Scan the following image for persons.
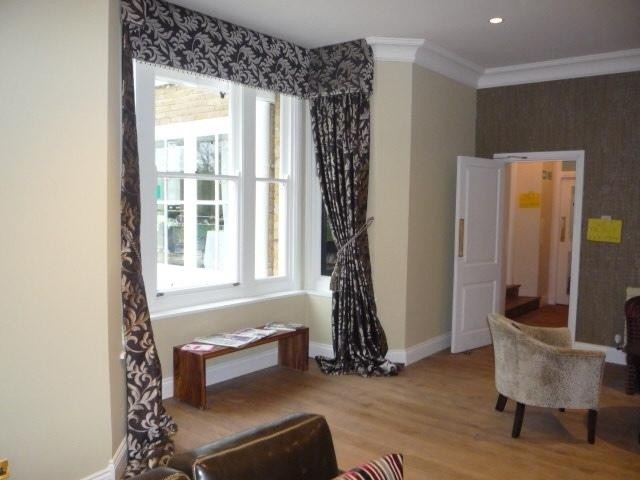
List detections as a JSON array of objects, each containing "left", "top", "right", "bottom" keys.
[{"left": 182, "top": 344, "right": 214, "bottom": 352}]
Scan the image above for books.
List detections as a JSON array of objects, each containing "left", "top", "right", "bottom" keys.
[{"left": 264, "top": 321, "right": 303, "bottom": 332}]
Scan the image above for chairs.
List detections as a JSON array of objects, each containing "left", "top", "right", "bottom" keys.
[{"left": 488, "top": 309, "right": 607, "bottom": 445}]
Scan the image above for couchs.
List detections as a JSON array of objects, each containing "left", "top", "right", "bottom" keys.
[{"left": 130, "top": 413, "right": 342, "bottom": 480}]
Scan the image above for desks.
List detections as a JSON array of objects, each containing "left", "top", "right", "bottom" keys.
[{"left": 176, "top": 324, "right": 311, "bottom": 409}]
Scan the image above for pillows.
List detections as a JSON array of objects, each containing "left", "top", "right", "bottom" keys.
[{"left": 330, "top": 450, "right": 404, "bottom": 480}]
